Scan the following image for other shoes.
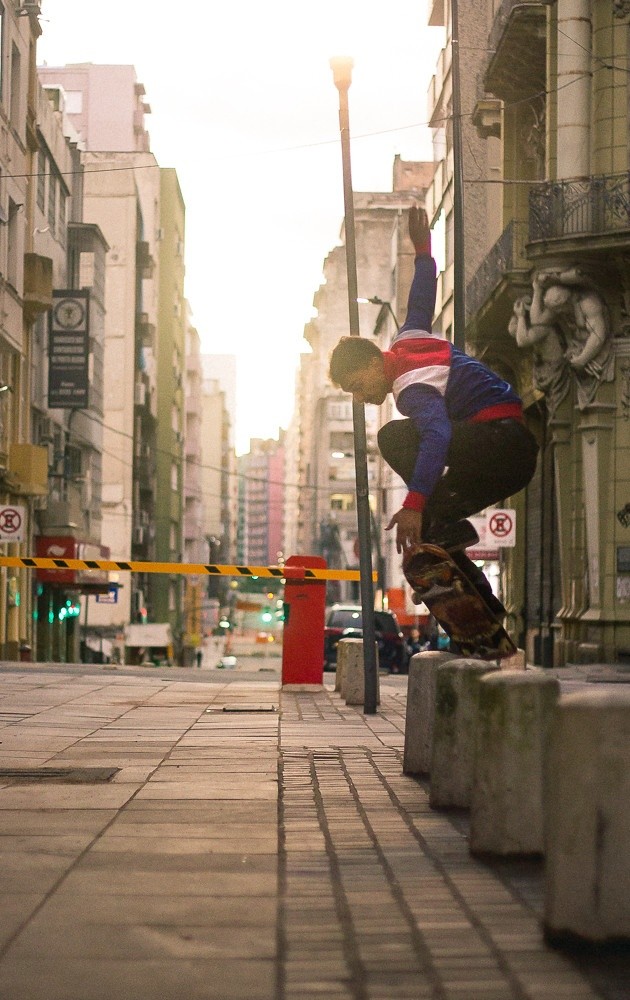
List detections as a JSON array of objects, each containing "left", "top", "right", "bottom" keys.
[
  {"left": 420, "top": 520, "right": 480, "bottom": 553},
  {"left": 483, "top": 594, "right": 507, "bottom": 617}
]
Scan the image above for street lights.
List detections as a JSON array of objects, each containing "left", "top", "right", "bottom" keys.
[{"left": 329, "top": 54, "right": 377, "bottom": 713}]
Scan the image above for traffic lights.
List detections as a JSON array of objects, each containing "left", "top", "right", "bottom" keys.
[{"left": 262, "top": 606, "right": 269, "bottom": 623}]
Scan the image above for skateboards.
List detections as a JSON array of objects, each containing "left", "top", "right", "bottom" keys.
[{"left": 402, "top": 543, "right": 517, "bottom": 661}]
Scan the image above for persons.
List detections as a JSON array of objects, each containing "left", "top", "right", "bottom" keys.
[
  {"left": 509, "top": 272, "right": 614, "bottom": 419},
  {"left": 329, "top": 202, "right": 540, "bottom": 661},
  {"left": 407, "top": 631, "right": 451, "bottom": 674},
  {"left": 196, "top": 651, "right": 202, "bottom": 668}
]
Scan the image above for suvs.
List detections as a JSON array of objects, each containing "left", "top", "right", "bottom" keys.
[{"left": 324, "top": 602, "right": 411, "bottom": 675}]
